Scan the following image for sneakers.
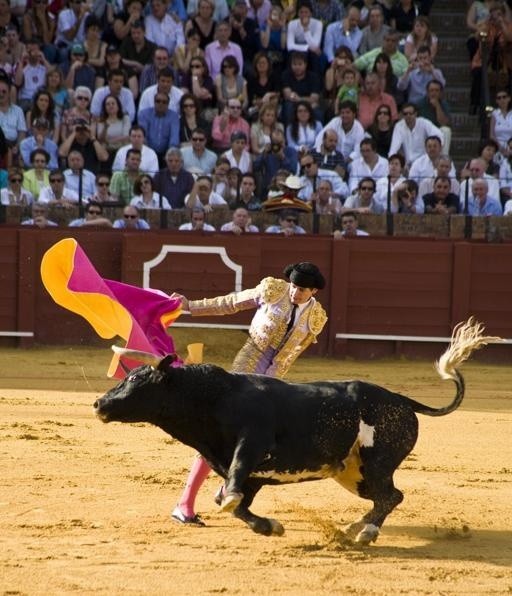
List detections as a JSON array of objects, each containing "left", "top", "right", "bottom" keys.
[
  {"left": 171, "top": 506, "right": 205, "bottom": 527},
  {"left": 215, "top": 487, "right": 224, "bottom": 505},
  {"left": 469, "top": 104, "right": 476, "bottom": 115}
]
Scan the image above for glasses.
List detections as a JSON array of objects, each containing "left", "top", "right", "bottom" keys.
[{"left": 13, "top": 67, "right": 413, "bottom": 217}]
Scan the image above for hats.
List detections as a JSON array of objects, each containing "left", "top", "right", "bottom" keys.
[
  {"left": 284, "top": 262, "right": 327, "bottom": 290},
  {"left": 72, "top": 44, "right": 85, "bottom": 55},
  {"left": 105, "top": 45, "right": 120, "bottom": 53}
]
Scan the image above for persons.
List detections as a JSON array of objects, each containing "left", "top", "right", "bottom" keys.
[
  {"left": 69, "top": 202, "right": 112, "bottom": 226},
  {"left": 113, "top": 206, "right": 149, "bottom": 229},
  {"left": 179, "top": 208, "right": 215, "bottom": 231},
  {"left": 170, "top": 262, "right": 328, "bottom": 527},
  {"left": 265, "top": 211, "right": 305, "bottom": 236},
  {"left": 221, "top": 208, "right": 259, "bottom": 234},
  {"left": 21, "top": 203, "right": 57, "bottom": 228},
  {"left": 334, "top": 212, "right": 368, "bottom": 240},
  {"left": 1, "top": 0, "right": 511, "bottom": 215}
]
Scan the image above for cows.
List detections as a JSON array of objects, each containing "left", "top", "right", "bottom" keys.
[{"left": 93, "top": 315, "right": 504, "bottom": 547}]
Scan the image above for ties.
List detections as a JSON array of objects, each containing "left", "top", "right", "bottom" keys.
[{"left": 286, "top": 303, "right": 299, "bottom": 332}]
